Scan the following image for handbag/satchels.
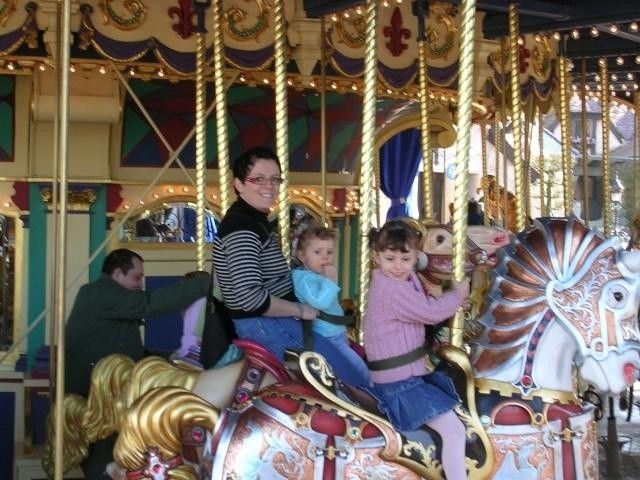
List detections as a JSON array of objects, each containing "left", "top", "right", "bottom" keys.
[{"left": 198, "top": 295, "right": 240, "bottom": 370}]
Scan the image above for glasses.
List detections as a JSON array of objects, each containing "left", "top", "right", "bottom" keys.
[{"left": 244, "top": 176, "right": 284, "bottom": 185}]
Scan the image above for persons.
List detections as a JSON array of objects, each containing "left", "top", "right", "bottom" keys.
[
  {"left": 212, "top": 143, "right": 372, "bottom": 389},
  {"left": 197, "top": 303, "right": 241, "bottom": 366},
  {"left": 56, "top": 249, "right": 209, "bottom": 480},
  {"left": 295, "top": 227, "right": 356, "bottom": 344},
  {"left": 364, "top": 221, "right": 472, "bottom": 479}
]
